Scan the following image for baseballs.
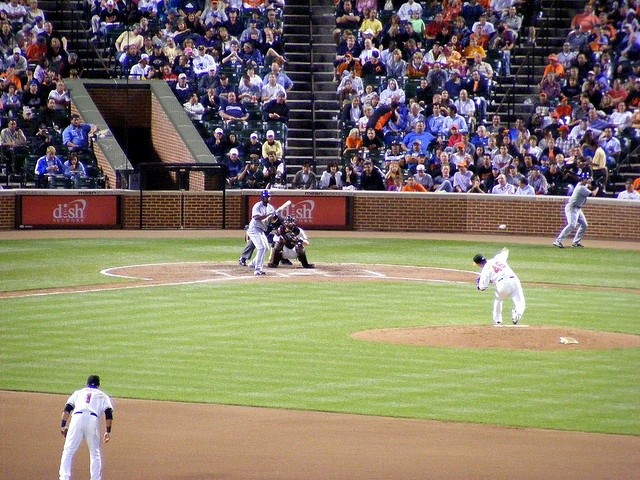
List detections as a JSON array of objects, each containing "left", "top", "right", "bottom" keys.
[{"left": 500, "top": 224, "right": 507, "bottom": 230}]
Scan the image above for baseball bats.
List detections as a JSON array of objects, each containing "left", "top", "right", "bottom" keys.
[{"left": 261, "top": 200, "right": 292, "bottom": 226}]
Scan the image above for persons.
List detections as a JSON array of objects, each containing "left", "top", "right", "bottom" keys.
[
  {"left": 551, "top": 170, "right": 600, "bottom": 249},
  {"left": 320, "top": 1, "right": 572, "bottom": 195},
  {"left": 57, "top": 375, "right": 114, "bottom": 480},
  {"left": 268, "top": 214, "right": 315, "bottom": 270},
  {"left": 246, "top": 190, "right": 276, "bottom": 276},
  {"left": 291, "top": 162, "right": 317, "bottom": 191},
  {"left": 0, "top": 0, "right": 89, "bottom": 188},
  {"left": 89, "top": 0, "right": 289, "bottom": 190},
  {"left": 616, "top": 178, "right": 640, "bottom": 201},
  {"left": 575, "top": 0, "right": 640, "bottom": 177},
  {"left": 238, "top": 209, "right": 293, "bottom": 267},
  {"left": 472, "top": 247, "right": 526, "bottom": 326}
]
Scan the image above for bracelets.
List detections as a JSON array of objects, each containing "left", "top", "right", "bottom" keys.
[
  {"left": 61, "top": 419, "right": 66, "bottom": 427},
  {"left": 106, "top": 426, "right": 111, "bottom": 433}
]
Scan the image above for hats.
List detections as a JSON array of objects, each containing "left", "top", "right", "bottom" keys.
[
  {"left": 138, "top": 53, "right": 149, "bottom": 62},
  {"left": 473, "top": 254, "right": 486, "bottom": 264},
  {"left": 229, "top": 147, "right": 239, "bottom": 155},
  {"left": 547, "top": 106, "right": 556, "bottom": 113},
  {"left": 580, "top": 171, "right": 590, "bottom": 179},
  {"left": 541, "top": 154, "right": 548, "bottom": 161},
  {"left": 457, "top": 162, "right": 466, "bottom": 167},
  {"left": 523, "top": 143, "right": 530, "bottom": 149},
  {"left": 34, "top": 16, "right": 42, "bottom": 22},
  {"left": 215, "top": 127, "right": 224, "bottom": 134},
  {"left": 106, "top": 0, "right": 114, "bottom": 6},
  {"left": 547, "top": 53, "right": 558, "bottom": 62},
  {"left": 391, "top": 140, "right": 399, "bottom": 145},
  {"left": 413, "top": 138, "right": 422, "bottom": 145},
  {"left": 250, "top": 133, "right": 258, "bottom": 139},
  {"left": 29, "top": 80, "right": 37, "bottom": 86},
  {"left": 457, "top": 143, "right": 465, "bottom": 149},
  {"left": 88, "top": 374, "right": 100, "bottom": 386},
  {"left": 556, "top": 125, "right": 570, "bottom": 131},
  {"left": 178, "top": 73, "right": 187, "bottom": 79},
  {"left": 13, "top": 47, "right": 22, "bottom": 54},
  {"left": 586, "top": 70, "right": 595, "bottom": 75},
  {"left": 492, "top": 165, "right": 500, "bottom": 170},
  {"left": 266, "top": 129, "right": 275, "bottom": 137},
  {"left": 450, "top": 124, "right": 459, "bottom": 130},
  {"left": 509, "top": 163, "right": 517, "bottom": 168},
  {"left": 416, "top": 164, "right": 426, "bottom": 171},
  {"left": 417, "top": 151, "right": 426, "bottom": 157}
]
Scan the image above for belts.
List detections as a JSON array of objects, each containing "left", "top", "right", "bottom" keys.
[
  {"left": 573, "top": 205, "right": 581, "bottom": 208},
  {"left": 498, "top": 276, "right": 514, "bottom": 282},
  {"left": 73, "top": 411, "right": 98, "bottom": 417}
]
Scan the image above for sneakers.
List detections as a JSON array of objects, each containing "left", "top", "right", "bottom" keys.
[
  {"left": 512, "top": 309, "right": 518, "bottom": 324},
  {"left": 248, "top": 262, "right": 255, "bottom": 269},
  {"left": 268, "top": 263, "right": 278, "bottom": 268},
  {"left": 303, "top": 263, "right": 315, "bottom": 269},
  {"left": 239, "top": 256, "right": 246, "bottom": 266},
  {"left": 493, "top": 321, "right": 502, "bottom": 325},
  {"left": 254, "top": 269, "right": 267, "bottom": 275},
  {"left": 283, "top": 260, "right": 292, "bottom": 265},
  {"left": 571, "top": 243, "right": 584, "bottom": 247},
  {"left": 553, "top": 241, "right": 564, "bottom": 248}
]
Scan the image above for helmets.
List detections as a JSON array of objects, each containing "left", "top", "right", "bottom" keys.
[
  {"left": 261, "top": 190, "right": 271, "bottom": 198},
  {"left": 272, "top": 215, "right": 283, "bottom": 230},
  {"left": 284, "top": 215, "right": 296, "bottom": 229}
]
